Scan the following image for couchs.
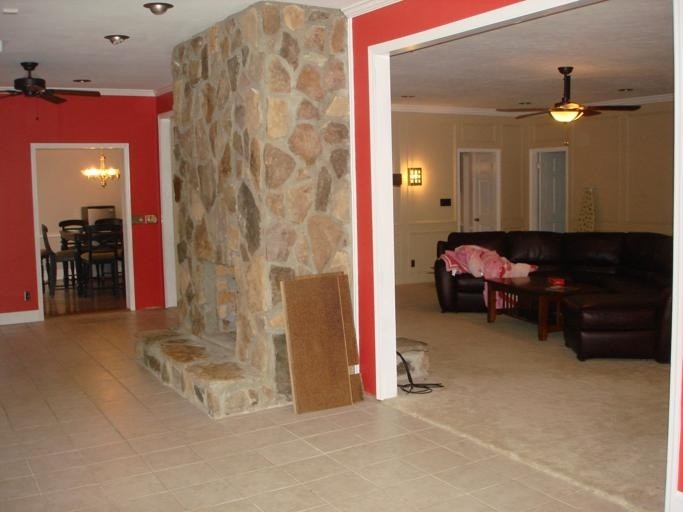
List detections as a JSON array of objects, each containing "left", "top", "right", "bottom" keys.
[{"left": 434, "top": 232, "right": 672, "bottom": 361}]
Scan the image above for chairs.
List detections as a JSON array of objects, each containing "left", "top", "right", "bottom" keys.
[{"left": 42, "top": 217, "right": 125, "bottom": 298}]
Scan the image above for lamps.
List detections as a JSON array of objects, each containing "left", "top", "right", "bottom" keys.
[
  {"left": 104, "top": 35, "right": 130, "bottom": 46},
  {"left": 549, "top": 97, "right": 587, "bottom": 123},
  {"left": 80, "top": 150, "right": 120, "bottom": 193},
  {"left": 143, "top": 2, "right": 174, "bottom": 16},
  {"left": 408, "top": 168, "right": 421, "bottom": 185}
]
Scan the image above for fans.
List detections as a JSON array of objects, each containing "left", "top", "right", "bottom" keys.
[
  {"left": 496, "top": 67, "right": 641, "bottom": 120},
  {"left": 0, "top": 62, "right": 100, "bottom": 104}
]
